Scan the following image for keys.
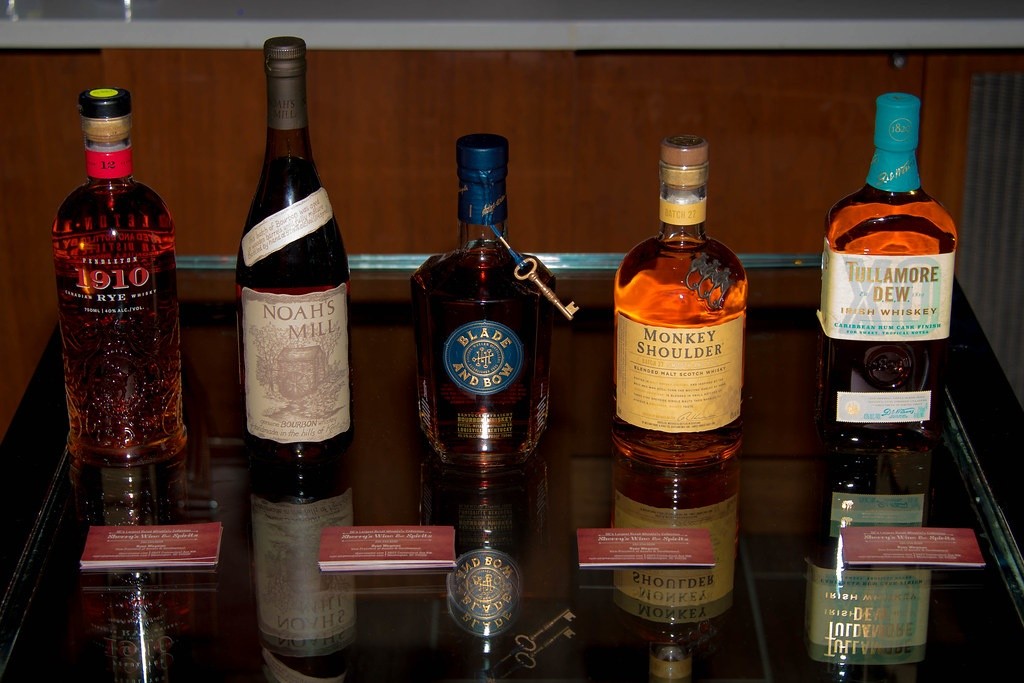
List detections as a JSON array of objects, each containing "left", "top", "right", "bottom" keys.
[
  {"left": 515, "top": 606, "right": 577, "bottom": 669},
  {"left": 514, "top": 257, "right": 579, "bottom": 321}
]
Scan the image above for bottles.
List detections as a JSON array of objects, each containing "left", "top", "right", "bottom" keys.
[
  {"left": 609, "top": 459, "right": 742, "bottom": 683},
  {"left": 804, "top": 446, "right": 935, "bottom": 683},
  {"left": 420, "top": 465, "right": 547, "bottom": 683},
  {"left": 237, "top": 35, "right": 349, "bottom": 464},
  {"left": 50, "top": 88, "right": 187, "bottom": 463},
  {"left": 614, "top": 131, "right": 748, "bottom": 470},
  {"left": 821, "top": 91, "right": 956, "bottom": 448},
  {"left": 71, "top": 465, "right": 193, "bottom": 683},
  {"left": 411, "top": 133, "right": 576, "bottom": 479},
  {"left": 251, "top": 465, "right": 356, "bottom": 683}
]
